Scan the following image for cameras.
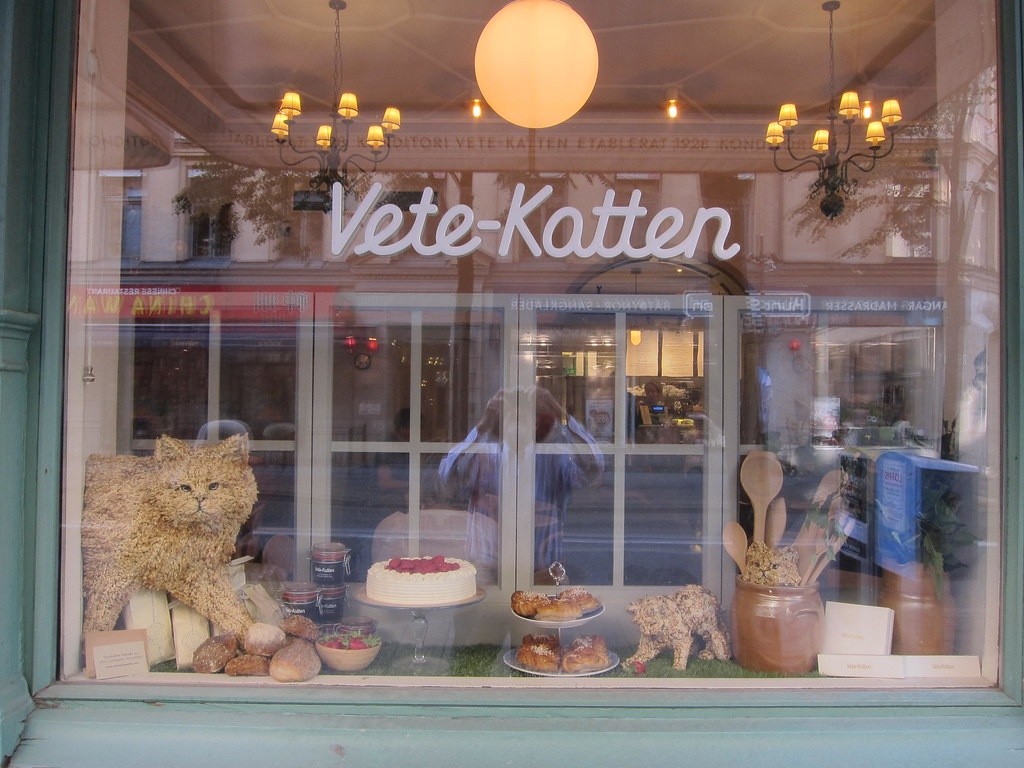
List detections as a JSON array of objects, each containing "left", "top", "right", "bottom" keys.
[{"left": 505, "top": 387, "right": 528, "bottom": 405}]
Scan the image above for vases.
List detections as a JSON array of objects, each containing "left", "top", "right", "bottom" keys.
[{"left": 730, "top": 574, "right": 825, "bottom": 672}]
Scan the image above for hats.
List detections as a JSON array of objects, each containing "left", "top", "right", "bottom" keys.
[{"left": 644, "top": 377, "right": 662, "bottom": 400}]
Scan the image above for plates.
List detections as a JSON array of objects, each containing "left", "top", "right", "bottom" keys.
[
  {"left": 511, "top": 594, "right": 605, "bottom": 628},
  {"left": 502, "top": 645, "right": 619, "bottom": 676}
]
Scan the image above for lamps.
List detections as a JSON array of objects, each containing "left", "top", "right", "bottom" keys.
[
  {"left": 763, "top": 0, "right": 905, "bottom": 219},
  {"left": 630, "top": 331, "right": 641, "bottom": 345},
  {"left": 475, "top": 0, "right": 599, "bottom": 128},
  {"left": 269, "top": 0, "right": 402, "bottom": 198}
]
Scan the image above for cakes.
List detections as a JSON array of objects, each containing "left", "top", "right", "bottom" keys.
[{"left": 366, "top": 556, "right": 477, "bottom": 606}]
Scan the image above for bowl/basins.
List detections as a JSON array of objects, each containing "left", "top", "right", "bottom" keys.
[{"left": 314, "top": 636, "right": 382, "bottom": 672}]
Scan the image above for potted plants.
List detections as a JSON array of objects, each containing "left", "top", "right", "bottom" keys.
[{"left": 872, "top": 472, "right": 984, "bottom": 658}]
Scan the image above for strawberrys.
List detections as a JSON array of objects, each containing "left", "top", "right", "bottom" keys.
[{"left": 319, "top": 633, "right": 373, "bottom": 649}]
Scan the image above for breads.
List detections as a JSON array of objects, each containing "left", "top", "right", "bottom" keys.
[
  {"left": 192, "top": 615, "right": 322, "bottom": 682},
  {"left": 516, "top": 634, "right": 613, "bottom": 673},
  {"left": 511, "top": 589, "right": 602, "bottom": 622}
]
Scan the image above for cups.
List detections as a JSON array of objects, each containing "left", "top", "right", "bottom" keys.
[
  {"left": 724, "top": 578, "right": 824, "bottom": 678},
  {"left": 333, "top": 616, "right": 379, "bottom": 635},
  {"left": 814, "top": 396, "right": 840, "bottom": 444}
]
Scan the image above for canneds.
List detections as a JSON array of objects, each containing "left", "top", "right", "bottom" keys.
[{"left": 275, "top": 542, "right": 378, "bottom": 633}]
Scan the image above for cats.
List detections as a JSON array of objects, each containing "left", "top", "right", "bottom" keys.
[
  {"left": 620, "top": 585, "right": 732, "bottom": 670},
  {"left": 82, "top": 431, "right": 258, "bottom": 641}
]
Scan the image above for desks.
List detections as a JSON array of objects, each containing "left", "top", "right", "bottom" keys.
[{"left": 852, "top": 444, "right": 939, "bottom": 523}]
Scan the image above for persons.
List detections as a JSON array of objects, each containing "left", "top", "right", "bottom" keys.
[
  {"left": 639, "top": 379, "right": 670, "bottom": 421},
  {"left": 370, "top": 411, "right": 428, "bottom": 520},
  {"left": 952, "top": 351, "right": 989, "bottom": 452},
  {"left": 197, "top": 362, "right": 788, "bottom": 705}
]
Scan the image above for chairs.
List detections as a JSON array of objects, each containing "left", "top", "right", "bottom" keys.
[{"left": 343, "top": 424, "right": 373, "bottom": 509}]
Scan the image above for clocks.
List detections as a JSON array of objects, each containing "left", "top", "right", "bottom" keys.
[{"left": 354, "top": 352, "right": 371, "bottom": 370}]
